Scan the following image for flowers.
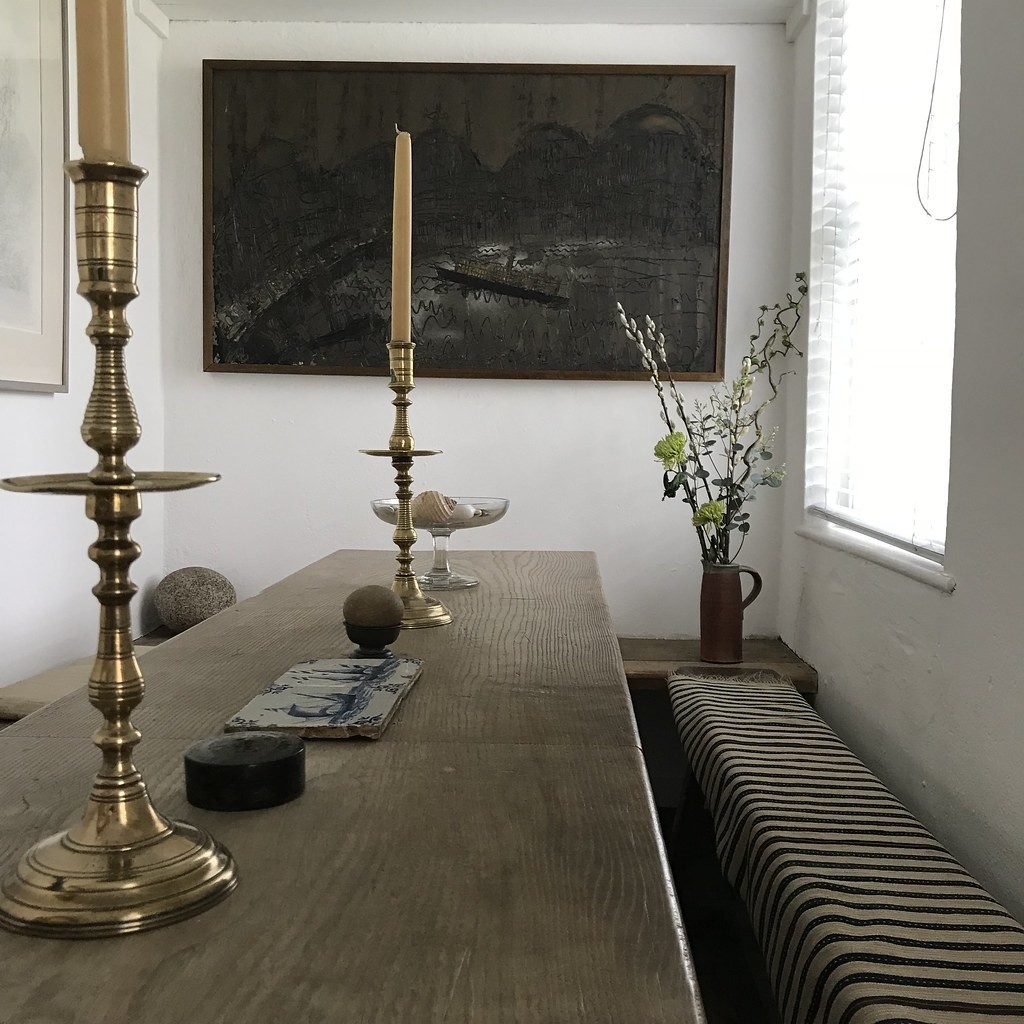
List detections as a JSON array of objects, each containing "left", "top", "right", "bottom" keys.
[{"left": 616, "top": 273, "right": 809, "bottom": 566}]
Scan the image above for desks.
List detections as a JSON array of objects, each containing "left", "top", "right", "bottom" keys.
[{"left": 0, "top": 548, "right": 704, "bottom": 1024}]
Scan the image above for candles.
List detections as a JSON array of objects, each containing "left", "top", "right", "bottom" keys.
[
  {"left": 74, "top": 0, "right": 131, "bottom": 162},
  {"left": 388, "top": 122, "right": 411, "bottom": 343}
]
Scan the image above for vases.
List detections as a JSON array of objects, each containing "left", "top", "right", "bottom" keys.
[{"left": 700, "top": 563, "right": 763, "bottom": 666}]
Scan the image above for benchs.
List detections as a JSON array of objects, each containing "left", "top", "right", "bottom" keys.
[
  {"left": 0, "top": 627, "right": 181, "bottom": 729},
  {"left": 667, "top": 663, "right": 1024, "bottom": 1024},
  {"left": 618, "top": 638, "right": 821, "bottom": 692}
]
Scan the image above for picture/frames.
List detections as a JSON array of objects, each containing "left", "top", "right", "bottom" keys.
[
  {"left": 201, "top": 60, "right": 735, "bottom": 383},
  {"left": 0, "top": 0, "right": 70, "bottom": 393}
]
